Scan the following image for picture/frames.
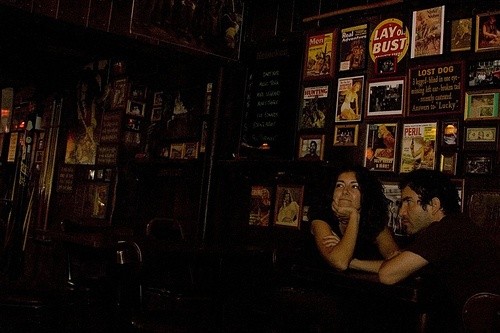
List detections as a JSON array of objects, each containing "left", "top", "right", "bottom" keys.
[
  {"left": 121, "top": 83, "right": 199, "bottom": 160},
  {"left": 272, "top": 3, "right": 500, "bottom": 241}
]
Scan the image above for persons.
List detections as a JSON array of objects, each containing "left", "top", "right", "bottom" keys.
[
  {"left": 306, "top": 161, "right": 401, "bottom": 333},
  {"left": 253, "top": 15, "right": 500, "bottom": 236},
  {"left": 379, "top": 169, "right": 500, "bottom": 333}
]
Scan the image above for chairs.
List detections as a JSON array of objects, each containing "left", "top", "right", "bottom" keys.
[{"left": 0, "top": 197, "right": 215, "bottom": 333}]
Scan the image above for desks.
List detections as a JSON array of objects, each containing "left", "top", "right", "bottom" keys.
[
  {"left": 31, "top": 227, "right": 260, "bottom": 333},
  {"left": 289, "top": 263, "right": 445, "bottom": 333}
]
